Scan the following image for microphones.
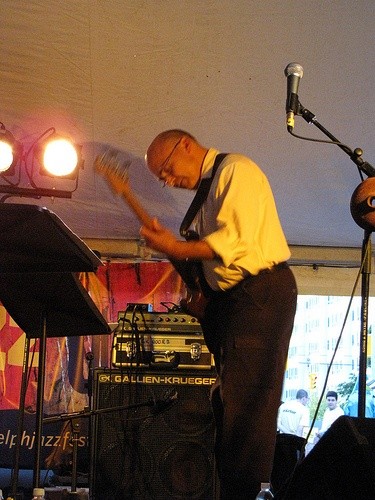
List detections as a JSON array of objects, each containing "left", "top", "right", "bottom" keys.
[{"left": 283, "top": 61, "right": 305, "bottom": 133}]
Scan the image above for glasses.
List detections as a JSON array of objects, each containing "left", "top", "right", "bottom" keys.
[{"left": 157, "top": 137, "right": 182, "bottom": 188}]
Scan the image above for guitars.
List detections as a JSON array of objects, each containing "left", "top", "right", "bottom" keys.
[{"left": 94, "top": 145, "right": 230, "bottom": 367}]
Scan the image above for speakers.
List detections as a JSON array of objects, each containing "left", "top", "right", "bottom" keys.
[
  {"left": 277, "top": 416, "right": 375, "bottom": 500},
  {"left": 90, "top": 367, "right": 219, "bottom": 500}
]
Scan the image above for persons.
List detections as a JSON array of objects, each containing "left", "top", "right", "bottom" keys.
[
  {"left": 314, "top": 391, "right": 344, "bottom": 442},
  {"left": 140, "top": 130, "right": 297, "bottom": 500},
  {"left": 276, "top": 389, "right": 310, "bottom": 439}
]
